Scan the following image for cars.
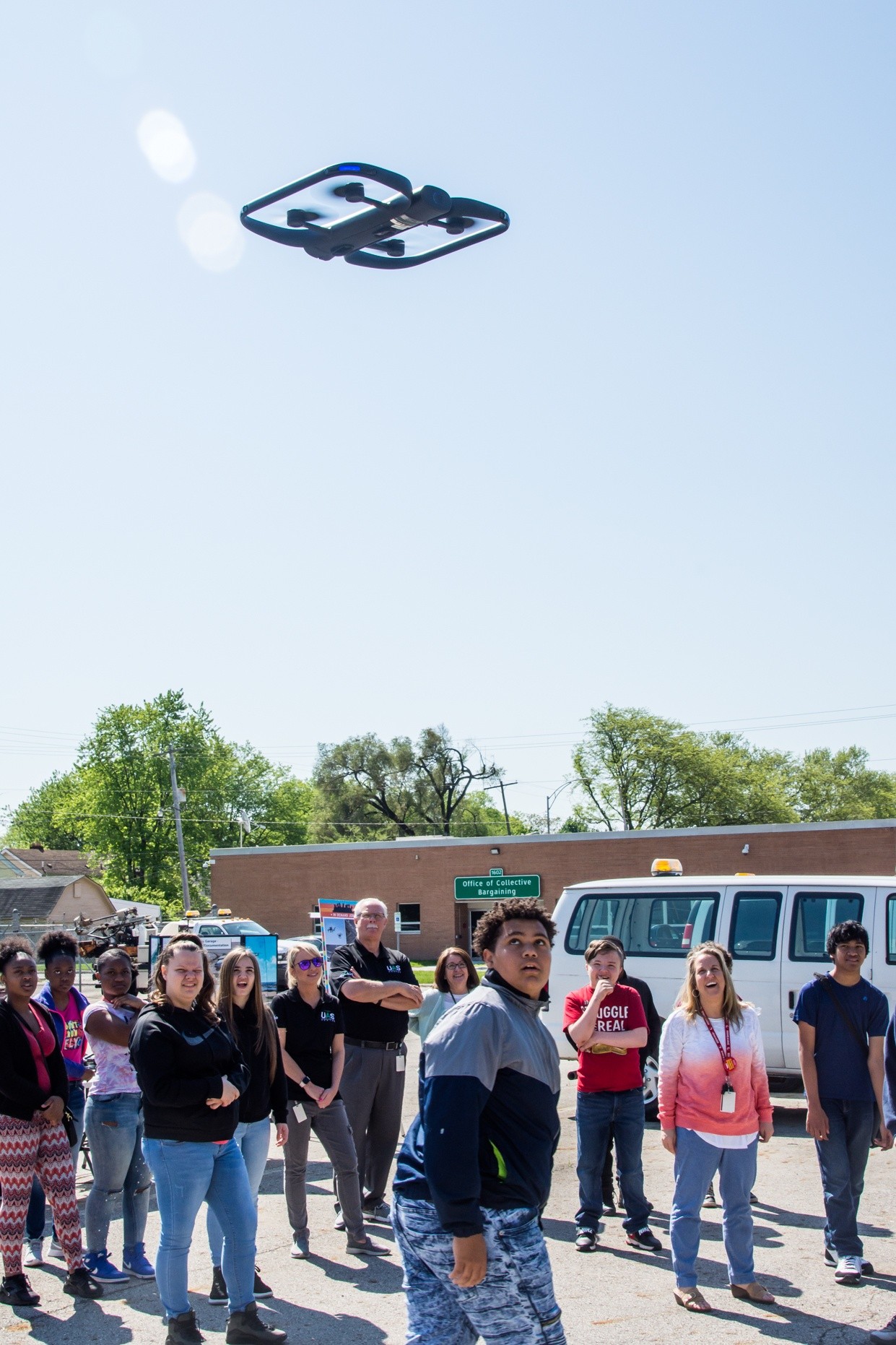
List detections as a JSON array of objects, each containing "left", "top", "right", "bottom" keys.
[{"left": 284, "top": 934, "right": 340, "bottom": 951}]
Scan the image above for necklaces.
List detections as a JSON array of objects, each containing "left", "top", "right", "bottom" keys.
[{"left": 449, "top": 989, "right": 470, "bottom": 1004}]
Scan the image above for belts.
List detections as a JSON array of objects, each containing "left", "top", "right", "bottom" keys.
[{"left": 344, "top": 1038, "right": 404, "bottom": 1050}]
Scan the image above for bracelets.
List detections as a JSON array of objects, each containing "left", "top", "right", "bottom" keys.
[{"left": 376, "top": 999, "right": 382, "bottom": 1006}]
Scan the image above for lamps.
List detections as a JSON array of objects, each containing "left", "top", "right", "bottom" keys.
[{"left": 491, "top": 848, "right": 499, "bottom": 854}]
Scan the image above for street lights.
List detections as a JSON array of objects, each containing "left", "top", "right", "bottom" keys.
[{"left": 546, "top": 776, "right": 589, "bottom": 834}]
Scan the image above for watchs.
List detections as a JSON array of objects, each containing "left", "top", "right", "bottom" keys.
[{"left": 299, "top": 1076, "right": 311, "bottom": 1088}]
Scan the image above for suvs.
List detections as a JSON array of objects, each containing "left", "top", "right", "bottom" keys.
[{"left": 159, "top": 909, "right": 324, "bottom": 991}]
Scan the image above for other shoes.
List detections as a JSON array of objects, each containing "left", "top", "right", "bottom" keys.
[
  {"left": 253, "top": 1264, "right": 273, "bottom": 1297},
  {"left": 870, "top": 1315, "right": 896, "bottom": 1345},
  {"left": 601, "top": 1191, "right": 618, "bottom": 1216},
  {"left": 346, "top": 1237, "right": 391, "bottom": 1255},
  {"left": 208, "top": 1266, "right": 228, "bottom": 1304},
  {"left": 362, "top": 1202, "right": 392, "bottom": 1222},
  {"left": 702, "top": 1182, "right": 717, "bottom": 1207},
  {"left": 618, "top": 1194, "right": 654, "bottom": 1209},
  {"left": 750, "top": 1192, "right": 758, "bottom": 1203},
  {"left": 334, "top": 1210, "right": 345, "bottom": 1229},
  {"left": 290, "top": 1237, "right": 310, "bottom": 1258}
]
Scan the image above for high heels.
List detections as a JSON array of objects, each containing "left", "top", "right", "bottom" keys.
[
  {"left": 730, "top": 1282, "right": 775, "bottom": 1304},
  {"left": 673, "top": 1287, "right": 712, "bottom": 1312}
]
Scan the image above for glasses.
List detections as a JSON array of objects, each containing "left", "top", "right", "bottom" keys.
[
  {"left": 292, "top": 957, "right": 323, "bottom": 971},
  {"left": 357, "top": 912, "right": 384, "bottom": 918},
  {"left": 446, "top": 961, "right": 465, "bottom": 968}
]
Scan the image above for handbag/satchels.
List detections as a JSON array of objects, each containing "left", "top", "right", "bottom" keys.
[{"left": 62, "top": 1108, "right": 79, "bottom": 1147}]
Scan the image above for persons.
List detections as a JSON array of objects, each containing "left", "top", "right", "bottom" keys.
[
  {"left": 656, "top": 941, "right": 776, "bottom": 1312},
  {"left": 270, "top": 943, "right": 391, "bottom": 1258},
  {"left": 206, "top": 945, "right": 289, "bottom": 1305},
  {"left": 407, "top": 947, "right": 480, "bottom": 1050},
  {"left": 328, "top": 898, "right": 424, "bottom": 1230},
  {"left": 389, "top": 898, "right": 568, "bottom": 1345},
  {"left": 127, "top": 933, "right": 288, "bottom": 1345},
  {"left": 870, "top": 1316, "right": 896, "bottom": 1345},
  {"left": 793, "top": 919, "right": 896, "bottom": 1283},
  {"left": 0, "top": 931, "right": 156, "bottom": 1306},
  {"left": 562, "top": 935, "right": 662, "bottom": 1251}
]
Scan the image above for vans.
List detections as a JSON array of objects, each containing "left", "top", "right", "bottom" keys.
[{"left": 549, "top": 857, "right": 896, "bottom": 1122}]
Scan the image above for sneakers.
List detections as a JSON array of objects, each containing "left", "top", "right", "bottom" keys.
[
  {"left": 0, "top": 1273, "right": 40, "bottom": 1305},
  {"left": 23, "top": 1234, "right": 45, "bottom": 1266},
  {"left": 576, "top": 1226, "right": 597, "bottom": 1251},
  {"left": 83, "top": 1249, "right": 131, "bottom": 1284},
  {"left": 165, "top": 1308, "right": 203, "bottom": 1345},
  {"left": 122, "top": 1242, "right": 155, "bottom": 1279},
  {"left": 225, "top": 1300, "right": 287, "bottom": 1343},
  {"left": 62, "top": 1267, "right": 104, "bottom": 1298},
  {"left": 626, "top": 1226, "right": 662, "bottom": 1250},
  {"left": 835, "top": 1255, "right": 862, "bottom": 1283},
  {"left": 824, "top": 1247, "right": 874, "bottom": 1275},
  {"left": 47, "top": 1238, "right": 87, "bottom": 1257}
]
